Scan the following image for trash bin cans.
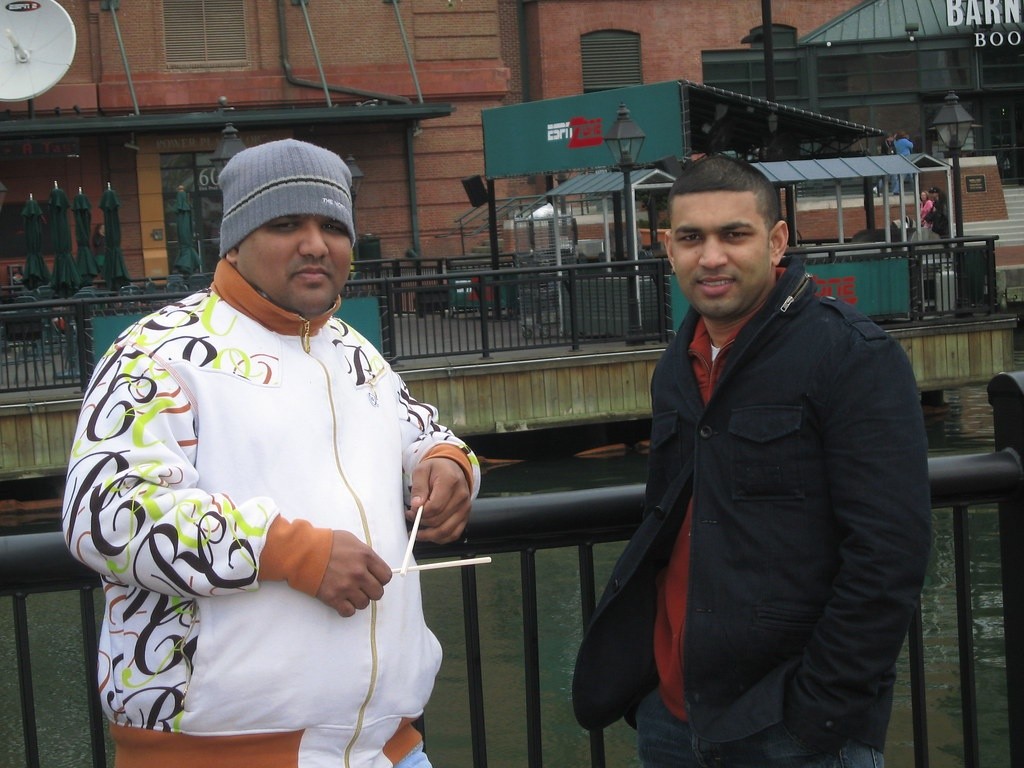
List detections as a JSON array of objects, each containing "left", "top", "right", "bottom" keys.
[
  {"left": 353, "top": 236, "right": 382, "bottom": 266},
  {"left": 953, "top": 250, "right": 990, "bottom": 310},
  {"left": 448, "top": 261, "right": 519, "bottom": 318}
]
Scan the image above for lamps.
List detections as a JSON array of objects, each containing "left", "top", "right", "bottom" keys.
[{"left": 903, "top": 22, "right": 919, "bottom": 36}]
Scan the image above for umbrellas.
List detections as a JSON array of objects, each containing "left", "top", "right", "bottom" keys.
[{"left": 20, "top": 180, "right": 202, "bottom": 380}]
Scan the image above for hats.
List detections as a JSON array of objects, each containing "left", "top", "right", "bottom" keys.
[{"left": 218, "top": 138, "right": 356, "bottom": 259}]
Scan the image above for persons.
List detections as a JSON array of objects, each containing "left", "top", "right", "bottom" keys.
[
  {"left": 572, "top": 150, "right": 932, "bottom": 768},
  {"left": 60, "top": 137, "right": 482, "bottom": 767},
  {"left": 916, "top": 186, "right": 951, "bottom": 258},
  {"left": 872, "top": 129, "right": 915, "bottom": 195}
]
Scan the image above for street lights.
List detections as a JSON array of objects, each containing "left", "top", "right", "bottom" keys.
[
  {"left": 933, "top": 91, "right": 975, "bottom": 320},
  {"left": 603, "top": 101, "right": 646, "bottom": 346}
]
